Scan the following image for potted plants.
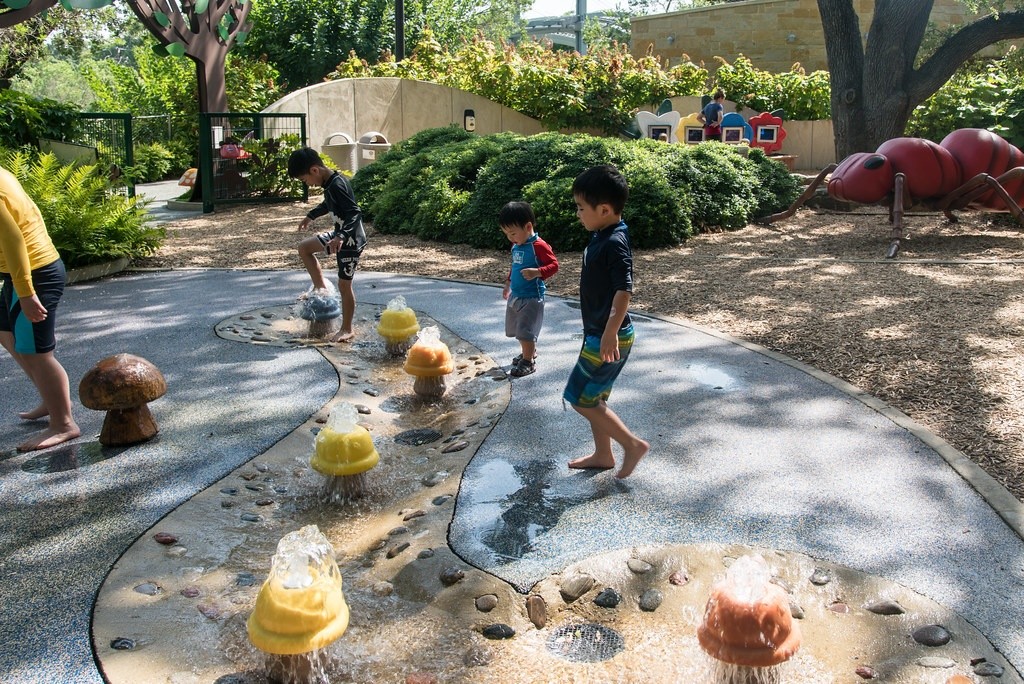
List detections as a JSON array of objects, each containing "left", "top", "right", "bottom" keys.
[{"left": 219, "top": 136, "right": 241, "bottom": 159}]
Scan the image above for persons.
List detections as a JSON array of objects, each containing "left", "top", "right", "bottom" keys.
[
  {"left": 739, "top": 138, "right": 751, "bottom": 158},
  {"left": 563, "top": 165, "right": 650, "bottom": 479},
  {"left": 658, "top": 133, "right": 668, "bottom": 143},
  {"left": 697, "top": 88, "right": 726, "bottom": 141},
  {"left": 498, "top": 200, "right": 560, "bottom": 377},
  {"left": 0, "top": 167, "right": 81, "bottom": 452},
  {"left": 288, "top": 147, "right": 367, "bottom": 340}
]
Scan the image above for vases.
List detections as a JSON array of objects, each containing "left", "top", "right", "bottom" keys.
[{"left": 771, "top": 155, "right": 798, "bottom": 172}]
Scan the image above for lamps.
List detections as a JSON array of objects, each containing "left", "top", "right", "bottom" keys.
[
  {"left": 666, "top": 36, "right": 673, "bottom": 45},
  {"left": 787, "top": 33, "right": 796, "bottom": 43}
]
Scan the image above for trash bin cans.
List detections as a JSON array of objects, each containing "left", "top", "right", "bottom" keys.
[
  {"left": 356, "top": 132, "right": 392, "bottom": 170},
  {"left": 321, "top": 132, "right": 357, "bottom": 176}
]
